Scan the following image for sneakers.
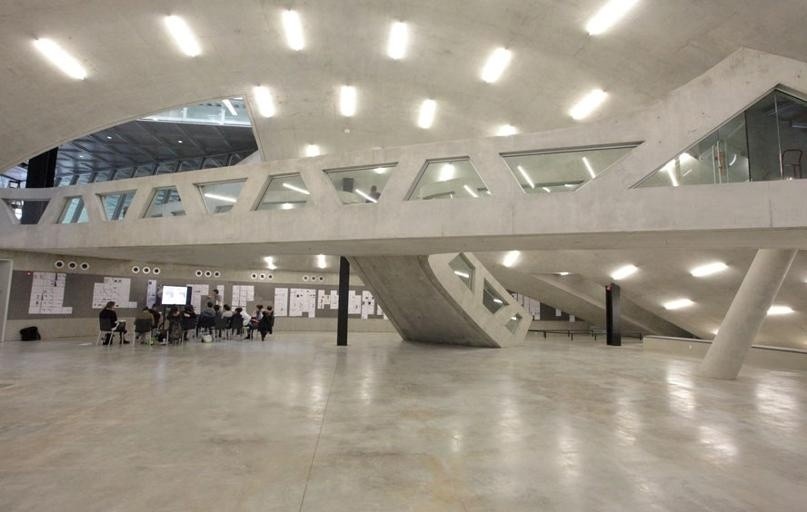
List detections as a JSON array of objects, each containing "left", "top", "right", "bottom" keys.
[{"left": 121, "top": 339, "right": 130, "bottom": 343}]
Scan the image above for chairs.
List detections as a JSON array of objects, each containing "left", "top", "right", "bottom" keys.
[
  {"left": 131, "top": 317, "right": 264, "bottom": 346},
  {"left": 95, "top": 317, "right": 125, "bottom": 346}
]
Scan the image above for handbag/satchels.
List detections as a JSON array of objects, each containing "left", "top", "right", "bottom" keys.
[
  {"left": 20, "top": 327, "right": 40, "bottom": 340},
  {"left": 202, "top": 334, "right": 212, "bottom": 343}
]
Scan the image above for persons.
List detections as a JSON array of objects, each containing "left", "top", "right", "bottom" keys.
[
  {"left": 133, "top": 289, "right": 274, "bottom": 346},
  {"left": 102, "top": 301, "right": 130, "bottom": 345},
  {"left": 365, "top": 185, "right": 381, "bottom": 203}
]
